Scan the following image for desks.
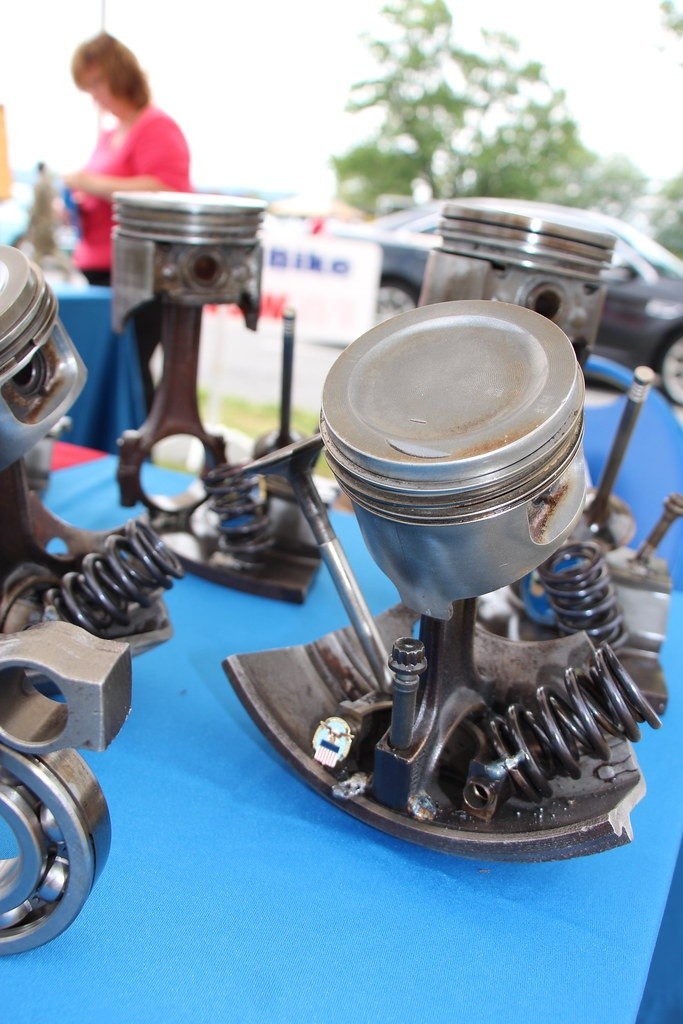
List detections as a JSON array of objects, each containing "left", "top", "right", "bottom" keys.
[{"left": 0, "top": 282, "right": 683, "bottom": 1024}]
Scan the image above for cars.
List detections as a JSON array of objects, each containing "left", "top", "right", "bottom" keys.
[{"left": 325, "top": 198, "right": 683, "bottom": 405}]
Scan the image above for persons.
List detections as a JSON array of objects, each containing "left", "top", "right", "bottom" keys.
[{"left": 65, "top": 32, "right": 192, "bottom": 403}]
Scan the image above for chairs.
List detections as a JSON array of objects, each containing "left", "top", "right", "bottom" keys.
[{"left": 568, "top": 355, "right": 683, "bottom": 589}]
